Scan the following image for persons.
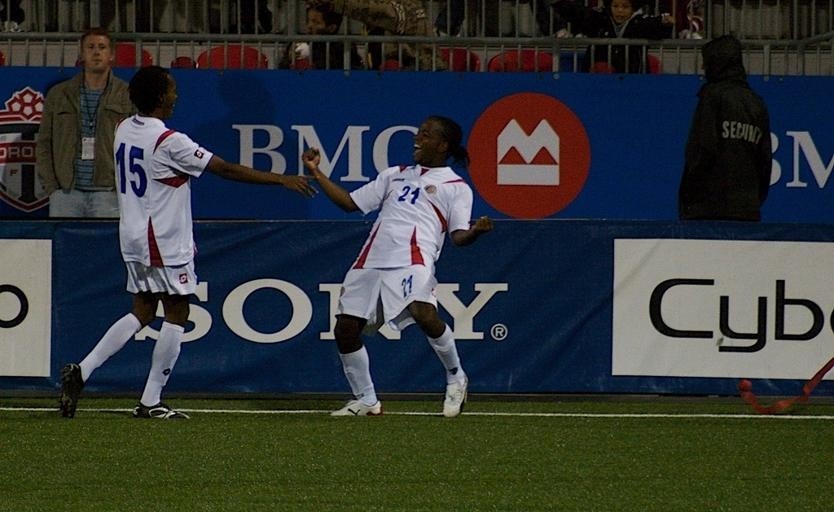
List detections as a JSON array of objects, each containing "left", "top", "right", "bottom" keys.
[
  {"left": 302, "top": 115, "right": 494, "bottom": 417},
  {"left": 679, "top": 33, "right": 772, "bottom": 220},
  {"left": 35, "top": 29, "right": 139, "bottom": 220},
  {"left": 59, "top": 65, "right": 320, "bottom": 419},
  {"left": 1, "top": 1, "right": 705, "bottom": 72}
]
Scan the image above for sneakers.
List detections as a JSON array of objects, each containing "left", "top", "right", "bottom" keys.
[
  {"left": 131, "top": 401, "right": 191, "bottom": 421},
  {"left": 442, "top": 375, "right": 470, "bottom": 419},
  {"left": 328, "top": 398, "right": 384, "bottom": 416},
  {"left": 59, "top": 362, "right": 85, "bottom": 420}
]
